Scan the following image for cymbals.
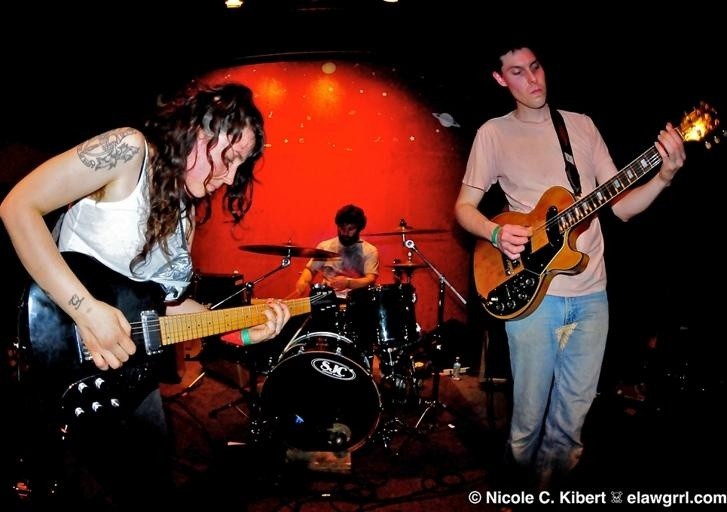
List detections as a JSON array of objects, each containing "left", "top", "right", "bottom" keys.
[
  {"left": 239, "top": 245, "right": 338, "bottom": 258},
  {"left": 360, "top": 230, "right": 448, "bottom": 236},
  {"left": 383, "top": 261, "right": 429, "bottom": 270}
]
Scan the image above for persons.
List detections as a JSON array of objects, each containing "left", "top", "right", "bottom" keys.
[
  {"left": 297, "top": 205, "right": 379, "bottom": 374},
  {"left": 0, "top": 80, "right": 291, "bottom": 512},
  {"left": 451, "top": 38, "right": 687, "bottom": 512}
]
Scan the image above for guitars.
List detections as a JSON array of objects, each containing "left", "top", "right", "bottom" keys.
[
  {"left": 16, "top": 248, "right": 340, "bottom": 426},
  {"left": 471, "top": 100, "right": 725, "bottom": 322}
]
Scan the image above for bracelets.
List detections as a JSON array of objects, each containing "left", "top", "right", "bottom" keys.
[
  {"left": 345, "top": 277, "right": 353, "bottom": 288},
  {"left": 489, "top": 226, "right": 501, "bottom": 248},
  {"left": 241, "top": 330, "right": 250, "bottom": 347}
]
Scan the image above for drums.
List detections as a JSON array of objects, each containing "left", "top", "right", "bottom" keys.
[
  {"left": 256, "top": 332, "right": 382, "bottom": 463},
  {"left": 346, "top": 282, "right": 424, "bottom": 360}
]
